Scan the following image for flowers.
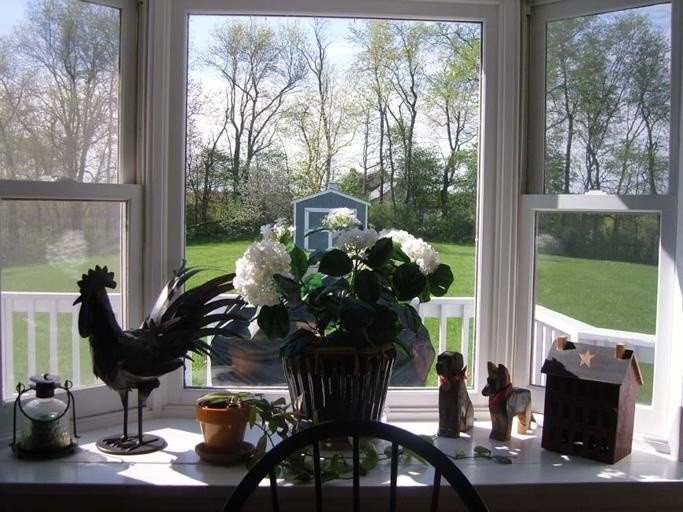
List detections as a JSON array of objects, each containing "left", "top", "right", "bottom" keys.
[{"left": 214, "top": 207, "right": 454, "bottom": 378}]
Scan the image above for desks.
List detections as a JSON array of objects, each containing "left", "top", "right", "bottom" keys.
[{"left": 1, "top": 395, "right": 683, "bottom": 512}]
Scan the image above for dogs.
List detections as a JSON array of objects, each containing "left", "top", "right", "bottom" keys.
[
  {"left": 435, "top": 350, "right": 474, "bottom": 438},
  {"left": 482, "top": 361, "right": 536, "bottom": 441}
]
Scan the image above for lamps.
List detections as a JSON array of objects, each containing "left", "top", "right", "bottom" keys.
[{"left": 9, "top": 373, "right": 81, "bottom": 460}]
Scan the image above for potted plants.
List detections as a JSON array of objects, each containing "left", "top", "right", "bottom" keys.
[{"left": 195, "top": 390, "right": 517, "bottom": 497}]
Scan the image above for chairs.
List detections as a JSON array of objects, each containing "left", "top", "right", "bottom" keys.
[{"left": 226, "top": 416, "right": 490, "bottom": 512}]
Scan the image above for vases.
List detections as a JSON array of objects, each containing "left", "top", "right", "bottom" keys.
[{"left": 279, "top": 346, "right": 397, "bottom": 443}]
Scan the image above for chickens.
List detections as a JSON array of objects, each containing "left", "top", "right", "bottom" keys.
[{"left": 72, "top": 258, "right": 249, "bottom": 452}]
